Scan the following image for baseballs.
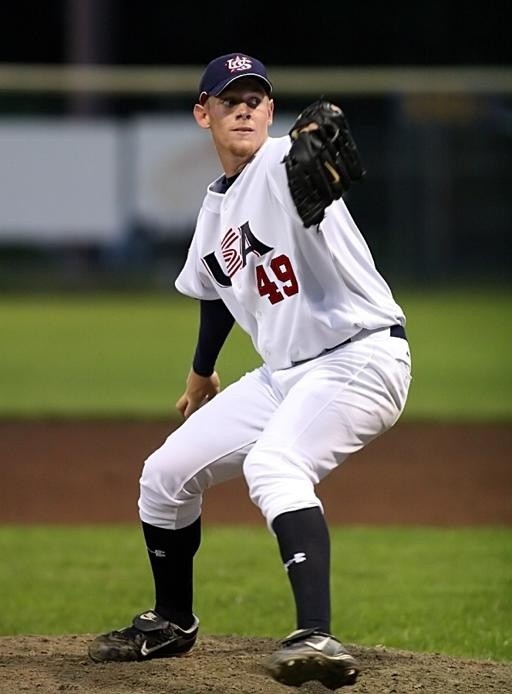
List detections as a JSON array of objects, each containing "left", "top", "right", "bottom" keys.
[{"left": 197, "top": 397, "right": 209, "bottom": 407}]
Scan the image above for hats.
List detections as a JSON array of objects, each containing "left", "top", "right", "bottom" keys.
[{"left": 197, "top": 52, "right": 273, "bottom": 106}]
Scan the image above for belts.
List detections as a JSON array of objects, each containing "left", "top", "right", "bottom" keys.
[{"left": 326, "top": 324, "right": 407, "bottom": 353}]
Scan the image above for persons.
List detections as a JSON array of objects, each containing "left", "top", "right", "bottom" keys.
[{"left": 89, "top": 50, "right": 416, "bottom": 686}]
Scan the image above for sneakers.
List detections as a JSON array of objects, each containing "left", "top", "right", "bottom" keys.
[
  {"left": 87, "top": 608, "right": 200, "bottom": 665},
  {"left": 268, "top": 627, "right": 361, "bottom": 691}
]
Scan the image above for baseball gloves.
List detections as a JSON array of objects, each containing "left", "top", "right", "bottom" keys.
[{"left": 281, "top": 101, "right": 368, "bottom": 234}]
